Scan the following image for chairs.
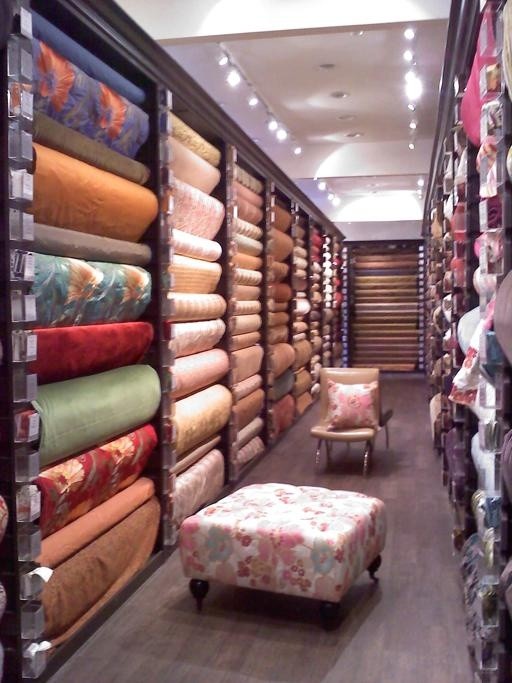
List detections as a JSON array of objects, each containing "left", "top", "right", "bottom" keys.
[{"left": 310, "top": 367, "right": 380, "bottom": 477}]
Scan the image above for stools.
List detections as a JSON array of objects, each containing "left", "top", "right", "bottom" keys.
[{"left": 179, "top": 482, "right": 388, "bottom": 633}]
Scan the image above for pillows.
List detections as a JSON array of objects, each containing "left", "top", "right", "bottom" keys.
[{"left": 324, "top": 377, "right": 382, "bottom": 432}]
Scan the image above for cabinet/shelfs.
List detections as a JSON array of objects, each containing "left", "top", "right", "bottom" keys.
[
  {"left": 421, "top": 1, "right": 512, "bottom": 682},
  {"left": 347, "top": 241, "right": 422, "bottom": 372},
  {"left": 0, "top": 0, "right": 346, "bottom": 683}
]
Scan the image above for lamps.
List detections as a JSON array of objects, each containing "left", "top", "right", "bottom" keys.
[
  {"left": 402, "top": 22, "right": 424, "bottom": 150},
  {"left": 212, "top": 41, "right": 343, "bottom": 209}
]
